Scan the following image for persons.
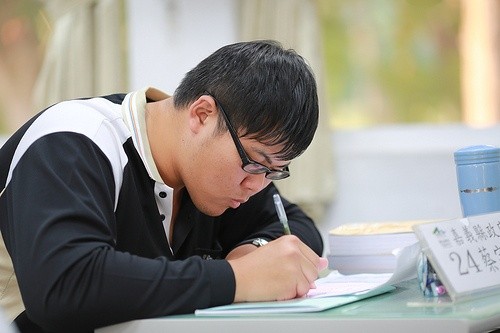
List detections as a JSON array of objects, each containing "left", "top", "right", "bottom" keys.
[{"left": 0, "top": 40, "right": 324, "bottom": 333}]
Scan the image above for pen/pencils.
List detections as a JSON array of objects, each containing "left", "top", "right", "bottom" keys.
[
  {"left": 436, "top": 285, "right": 445, "bottom": 295},
  {"left": 273, "top": 194, "right": 309, "bottom": 298}
]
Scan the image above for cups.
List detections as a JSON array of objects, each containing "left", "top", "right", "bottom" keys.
[{"left": 454, "top": 145, "right": 500, "bottom": 218}]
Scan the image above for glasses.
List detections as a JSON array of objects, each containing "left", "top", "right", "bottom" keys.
[{"left": 204, "top": 90, "right": 290, "bottom": 180}]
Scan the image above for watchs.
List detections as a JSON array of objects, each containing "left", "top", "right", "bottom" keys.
[{"left": 230, "top": 238, "right": 269, "bottom": 248}]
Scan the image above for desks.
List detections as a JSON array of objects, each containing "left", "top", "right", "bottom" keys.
[{"left": 90, "top": 275, "right": 500, "bottom": 333}]
